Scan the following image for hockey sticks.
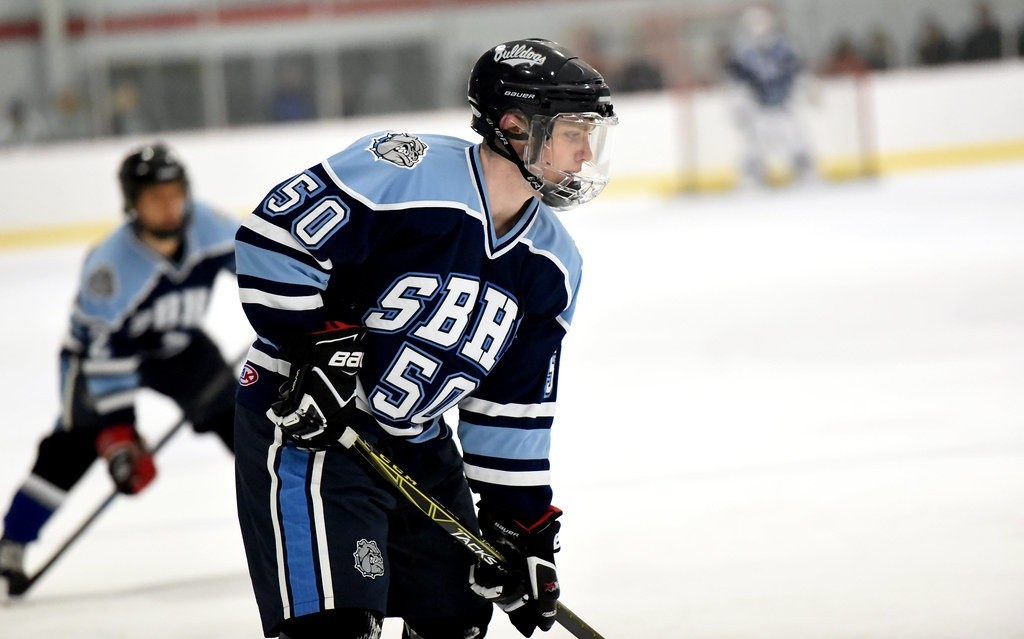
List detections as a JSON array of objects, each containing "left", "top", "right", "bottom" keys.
[
  {"left": 273, "top": 381, "right": 605, "bottom": 639},
  {"left": 17, "top": 343, "right": 252, "bottom": 588}
]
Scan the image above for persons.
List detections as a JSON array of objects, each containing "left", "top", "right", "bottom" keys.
[
  {"left": 818, "top": 1, "right": 1024, "bottom": 74},
  {"left": 110, "top": 75, "right": 165, "bottom": 136},
  {"left": 562, "top": 24, "right": 680, "bottom": 94},
  {"left": 232, "top": 38, "right": 621, "bottom": 639},
  {"left": 725, "top": 4, "right": 862, "bottom": 190},
  {"left": 0, "top": 143, "right": 242, "bottom": 597},
  {"left": 268, "top": 59, "right": 322, "bottom": 121}
]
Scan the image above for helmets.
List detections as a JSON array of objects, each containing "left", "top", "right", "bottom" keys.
[
  {"left": 466, "top": 37, "right": 618, "bottom": 210},
  {"left": 120, "top": 147, "right": 186, "bottom": 212}
]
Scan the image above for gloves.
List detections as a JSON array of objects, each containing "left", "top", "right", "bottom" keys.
[
  {"left": 266, "top": 320, "right": 368, "bottom": 454},
  {"left": 469, "top": 499, "right": 563, "bottom": 639},
  {"left": 97, "top": 426, "right": 157, "bottom": 494}
]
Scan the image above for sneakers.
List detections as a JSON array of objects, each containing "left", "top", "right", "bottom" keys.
[{"left": 0, "top": 540, "right": 30, "bottom": 604}]
